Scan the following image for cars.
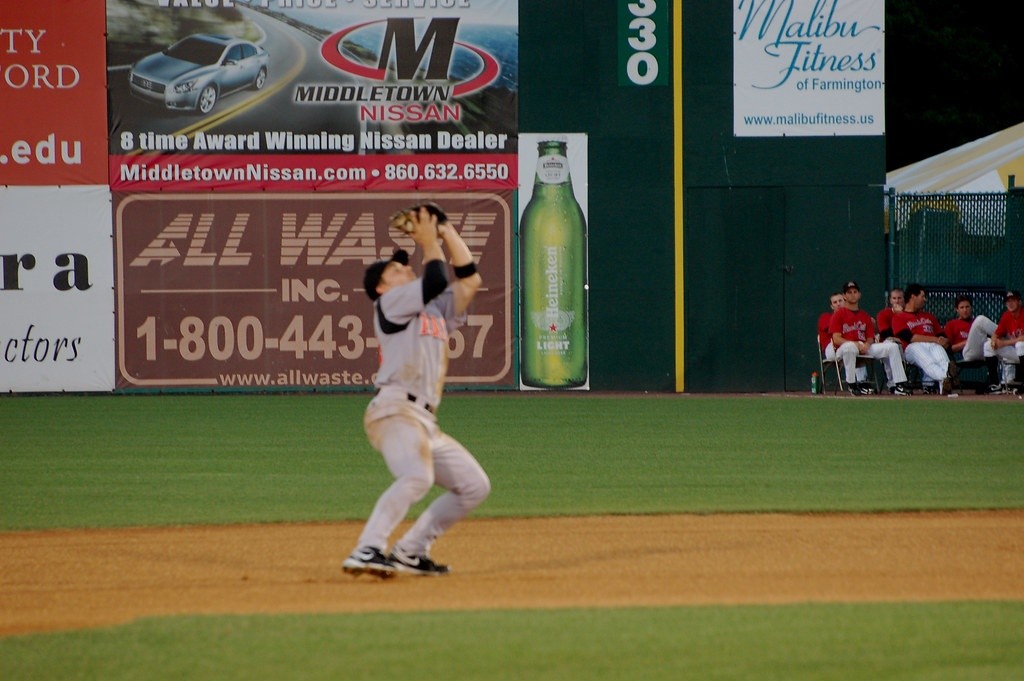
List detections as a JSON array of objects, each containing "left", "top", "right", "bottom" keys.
[{"left": 132, "top": 31, "right": 273, "bottom": 117}]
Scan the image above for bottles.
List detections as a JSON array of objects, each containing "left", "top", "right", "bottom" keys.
[
  {"left": 811, "top": 372, "right": 817, "bottom": 394},
  {"left": 520, "top": 140, "right": 588, "bottom": 387}
]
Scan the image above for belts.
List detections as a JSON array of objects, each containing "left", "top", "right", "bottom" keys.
[{"left": 376, "top": 389, "right": 432, "bottom": 413}]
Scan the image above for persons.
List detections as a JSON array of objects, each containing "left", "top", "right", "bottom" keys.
[
  {"left": 342, "top": 209, "right": 491, "bottom": 582},
  {"left": 818, "top": 282, "right": 1024, "bottom": 398}
]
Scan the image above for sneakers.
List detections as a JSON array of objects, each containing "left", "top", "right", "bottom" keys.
[
  {"left": 341, "top": 546, "right": 396, "bottom": 578},
  {"left": 388, "top": 550, "right": 449, "bottom": 574},
  {"left": 847, "top": 376, "right": 1020, "bottom": 396}
]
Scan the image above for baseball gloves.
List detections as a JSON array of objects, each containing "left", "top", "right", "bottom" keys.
[{"left": 387, "top": 203, "right": 449, "bottom": 235}]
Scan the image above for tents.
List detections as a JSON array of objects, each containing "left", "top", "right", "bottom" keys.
[{"left": 885, "top": 122, "right": 1024, "bottom": 236}]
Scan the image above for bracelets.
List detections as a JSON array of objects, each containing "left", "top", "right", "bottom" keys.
[{"left": 453, "top": 263, "right": 477, "bottom": 279}]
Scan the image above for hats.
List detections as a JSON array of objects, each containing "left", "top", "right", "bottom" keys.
[
  {"left": 842, "top": 281, "right": 860, "bottom": 294},
  {"left": 1003, "top": 290, "right": 1018, "bottom": 302},
  {"left": 363, "top": 249, "right": 409, "bottom": 301}
]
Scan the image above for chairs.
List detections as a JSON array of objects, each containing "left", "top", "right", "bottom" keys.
[
  {"left": 898, "top": 343, "right": 957, "bottom": 396},
  {"left": 817, "top": 335, "right": 872, "bottom": 395},
  {"left": 954, "top": 352, "right": 994, "bottom": 395},
  {"left": 831, "top": 338, "right": 880, "bottom": 396},
  {"left": 996, "top": 355, "right": 1024, "bottom": 396}
]
